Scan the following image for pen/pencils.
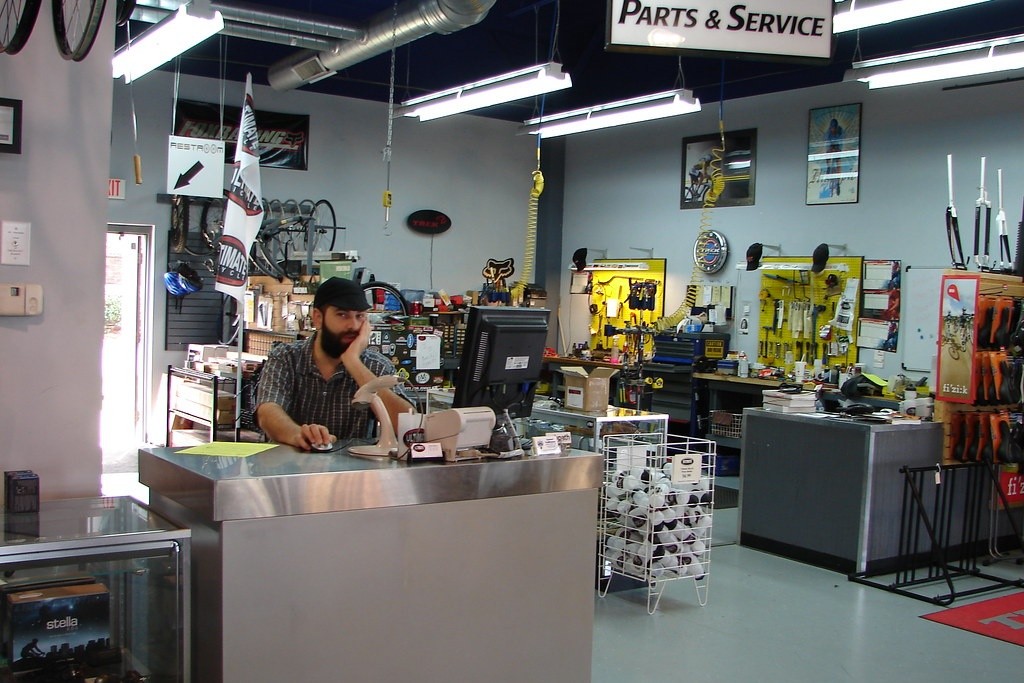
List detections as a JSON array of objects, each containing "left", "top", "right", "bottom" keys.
[{"left": 501, "top": 409, "right": 522, "bottom": 450}]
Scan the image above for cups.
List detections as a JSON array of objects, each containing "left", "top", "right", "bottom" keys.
[
  {"left": 409, "top": 301, "right": 425, "bottom": 317},
  {"left": 795, "top": 362, "right": 806, "bottom": 381}
]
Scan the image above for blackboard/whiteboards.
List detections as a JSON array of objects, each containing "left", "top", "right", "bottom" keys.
[
  {"left": 730, "top": 263, "right": 762, "bottom": 363},
  {"left": 902, "top": 265, "right": 952, "bottom": 372}
]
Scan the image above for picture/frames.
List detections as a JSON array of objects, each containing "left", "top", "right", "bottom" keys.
[
  {"left": 0, "top": 97, "right": 22, "bottom": 154},
  {"left": 680, "top": 127, "right": 758, "bottom": 209},
  {"left": 172, "top": 98, "right": 310, "bottom": 170},
  {"left": 805, "top": 102, "right": 860, "bottom": 206}
]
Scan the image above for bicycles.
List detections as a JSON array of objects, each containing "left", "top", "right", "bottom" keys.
[
  {"left": 171, "top": 194, "right": 409, "bottom": 346},
  {"left": 685, "top": 174, "right": 713, "bottom": 202},
  {"left": 827, "top": 148, "right": 839, "bottom": 196}
]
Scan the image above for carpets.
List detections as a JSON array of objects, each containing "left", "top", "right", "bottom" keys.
[{"left": 918, "top": 592, "right": 1024, "bottom": 647}]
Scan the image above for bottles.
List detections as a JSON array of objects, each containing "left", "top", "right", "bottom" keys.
[
  {"left": 738, "top": 351, "right": 749, "bottom": 378},
  {"left": 904, "top": 383, "right": 918, "bottom": 416},
  {"left": 603, "top": 466, "right": 712, "bottom": 584}
]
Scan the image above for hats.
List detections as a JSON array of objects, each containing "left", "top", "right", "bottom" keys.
[{"left": 314, "top": 276, "right": 372, "bottom": 311}]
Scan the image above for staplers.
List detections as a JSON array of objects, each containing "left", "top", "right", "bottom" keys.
[{"left": 779, "top": 383, "right": 802, "bottom": 394}]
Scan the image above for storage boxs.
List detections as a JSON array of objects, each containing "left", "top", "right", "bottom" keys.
[
  {"left": 169, "top": 377, "right": 264, "bottom": 445},
  {"left": 707, "top": 454, "right": 740, "bottom": 477},
  {"left": 557, "top": 364, "right": 620, "bottom": 412}
]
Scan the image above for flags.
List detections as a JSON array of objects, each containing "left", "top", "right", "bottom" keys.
[{"left": 214, "top": 75, "right": 264, "bottom": 303}]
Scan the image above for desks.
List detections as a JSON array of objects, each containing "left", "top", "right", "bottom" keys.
[{"left": 742, "top": 405, "right": 1023, "bottom": 578}]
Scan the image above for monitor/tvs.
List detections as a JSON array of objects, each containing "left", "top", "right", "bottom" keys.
[{"left": 451, "top": 306, "right": 551, "bottom": 450}]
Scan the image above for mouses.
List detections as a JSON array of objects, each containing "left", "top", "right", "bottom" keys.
[{"left": 305, "top": 440, "right": 333, "bottom": 452}]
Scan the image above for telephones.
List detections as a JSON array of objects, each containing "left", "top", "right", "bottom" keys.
[{"left": 843, "top": 403, "right": 874, "bottom": 416}]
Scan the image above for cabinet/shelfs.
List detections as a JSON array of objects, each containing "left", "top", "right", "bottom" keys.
[
  {"left": 235, "top": 289, "right": 314, "bottom": 444},
  {"left": 166, "top": 365, "right": 251, "bottom": 448},
  {"left": 546, "top": 328, "right": 820, "bottom": 453}
]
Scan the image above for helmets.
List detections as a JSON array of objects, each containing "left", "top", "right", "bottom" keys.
[{"left": 164, "top": 265, "right": 204, "bottom": 300}]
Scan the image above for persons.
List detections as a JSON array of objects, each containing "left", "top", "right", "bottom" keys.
[
  {"left": 946, "top": 307, "right": 969, "bottom": 335},
  {"left": 688, "top": 153, "right": 713, "bottom": 197},
  {"left": 824, "top": 119, "right": 844, "bottom": 196},
  {"left": 251, "top": 277, "right": 417, "bottom": 450}
]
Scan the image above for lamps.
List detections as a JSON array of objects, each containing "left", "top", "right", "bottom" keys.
[
  {"left": 832, "top": 0, "right": 989, "bottom": 35},
  {"left": 391, "top": 61, "right": 572, "bottom": 122},
  {"left": 842, "top": 33, "right": 1024, "bottom": 89},
  {"left": 517, "top": 90, "right": 701, "bottom": 138},
  {"left": 111, "top": 0, "right": 224, "bottom": 85}
]
